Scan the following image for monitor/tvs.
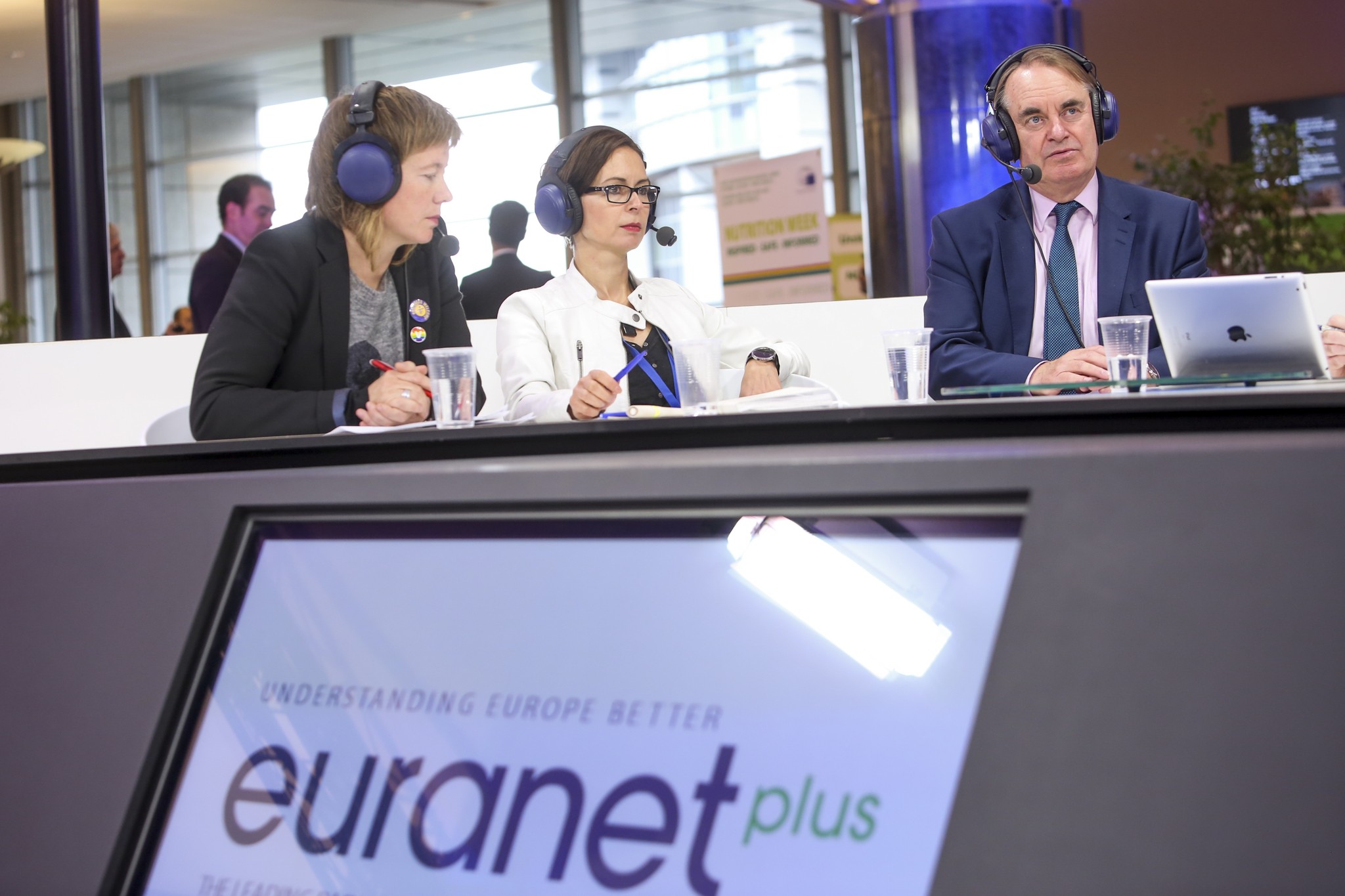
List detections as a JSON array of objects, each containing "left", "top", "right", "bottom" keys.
[
  {"left": 119, "top": 501, "right": 1021, "bottom": 896},
  {"left": 1226, "top": 92, "right": 1344, "bottom": 190}
]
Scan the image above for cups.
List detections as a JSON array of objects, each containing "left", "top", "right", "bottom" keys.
[
  {"left": 421, "top": 345, "right": 484, "bottom": 430},
  {"left": 1096, "top": 314, "right": 1152, "bottom": 395},
  {"left": 666, "top": 336, "right": 723, "bottom": 417},
  {"left": 881, "top": 326, "right": 935, "bottom": 404}
]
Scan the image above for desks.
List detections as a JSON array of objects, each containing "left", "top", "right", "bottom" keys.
[{"left": 0, "top": 374, "right": 1345, "bottom": 896}]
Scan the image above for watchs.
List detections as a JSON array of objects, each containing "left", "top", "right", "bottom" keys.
[
  {"left": 1145, "top": 359, "right": 1161, "bottom": 389},
  {"left": 745, "top": 347, "right": 780, "bottom": 376}
]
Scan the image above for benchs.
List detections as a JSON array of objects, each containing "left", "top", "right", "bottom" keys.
[{"left": 0, "top": 269, "right": 1345, "bottom": 468}]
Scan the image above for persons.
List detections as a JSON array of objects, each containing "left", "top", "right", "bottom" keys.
[
  {"left": 459, "top": 200, "right": 558, "bottom": 321},
  {"left": 1319, "top": 314, "right": 1345, "bottom": 379},
  {"left": 161, "top": 307, "right": 197, "bottom": 338},
  {"left": 54, "top": 222, "right": 132, "bottom": 339},
  {"left": 496, "top": 124, "right": 812, "bottom": 425},
  {"left": 922, "top": 44, "right": 1217, "bottom": 401},
  {"left": 187, "top": 88, "right": 488, "bottom": 441},
  {"left": 188, "top": 173, "right": 277, "bottom": 335}
]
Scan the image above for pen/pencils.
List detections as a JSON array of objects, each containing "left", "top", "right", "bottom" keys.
[
  {"left": 599, "top": 411, "right": 628, "bottom": 419},
  {"left": 611, "top": 349, "right": 650, "bottom": 382},
  {"left": 369, "top": 357, "right": 432, "bottom": 400}
]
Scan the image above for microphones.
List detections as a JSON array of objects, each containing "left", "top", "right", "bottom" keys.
[
  {"left": 656, "top": 226, "right": 677, "bottom": 246},
  {"left": 438, "top": 235, "right": 459, "bottom": 257},
  {"left": 1022, "top": 164, "right": 1042, "bottom": 184}
]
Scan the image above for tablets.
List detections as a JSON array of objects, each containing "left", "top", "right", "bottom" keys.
[{"left": 1144, "top": 273, "right": 1328, "bottom": 378}]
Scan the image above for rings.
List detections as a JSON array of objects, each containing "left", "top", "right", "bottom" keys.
[{"left": 401, "top": 388, "right": 411, "bottom": 399}]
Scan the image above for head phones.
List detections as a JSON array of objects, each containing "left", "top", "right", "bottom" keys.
[
  {"left": 980, "top": 45, "right": 1119, "bottom": 162},
  {"left": 329, "top": 78, "right": 402, "bottom": 208},
  {"left": 535, "top": 126, "right": 657, "bottom": 237}
]
